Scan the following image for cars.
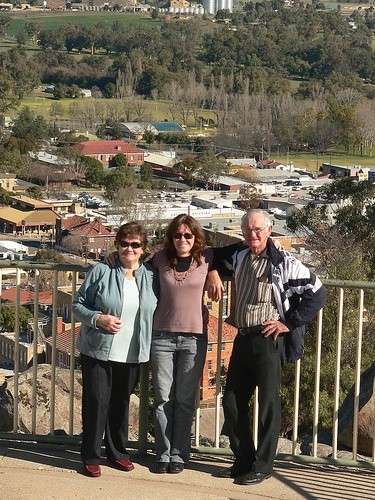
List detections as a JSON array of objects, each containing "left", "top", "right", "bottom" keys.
[
  {"left": 274, "top": 178, "right": 318, "bottom": 201},
  {"left": 74, "top": 191, "right": 111, "bottom": 208},
  {"left": 137, "top": 189, "right": 190, "bottom": 204}
]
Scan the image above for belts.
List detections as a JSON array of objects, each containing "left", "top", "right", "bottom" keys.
[{"left": 238, "top": 325, "right": 263, "bottom": 336}]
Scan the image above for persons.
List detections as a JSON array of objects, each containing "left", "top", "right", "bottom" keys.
[
  {"left": 105, "top": 213, "right": 285, "bottom": 473},
  {"left": 201, "top": 209, "right": 326, "bottom": 485},
  {"left": 71, "top": 220, "right": 157, "bottom": 478}
]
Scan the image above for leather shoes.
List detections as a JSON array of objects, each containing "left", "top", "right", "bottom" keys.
[
  {"left": 240, "top": 472, "right": 272, "bottom": 484},
  {"left": 220, "top": 465, "right": 247, "bottom": 478},
  {"left": 155, "top": 462, "right": 170, "bottom": 473},
  {"left": 169, "top": 462, "right": 184, "bottom": 473}
]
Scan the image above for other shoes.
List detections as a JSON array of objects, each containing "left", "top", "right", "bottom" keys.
[
  {"left": 113, "top": 459, "right": 133, "bottom": 471},
  {"left": 86, "top": 464, "right": 101, "bottom": 477}
]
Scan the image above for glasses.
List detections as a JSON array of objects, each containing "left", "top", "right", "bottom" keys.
[
  {"left": 119, "top": 241, "right": 142, "bottom": 249},
  {"left": 244, "top": 226, "right": 268, "bottom": 234},
  {"left": 174, "top": 233, "right": 194, "bottom": 239}
]
